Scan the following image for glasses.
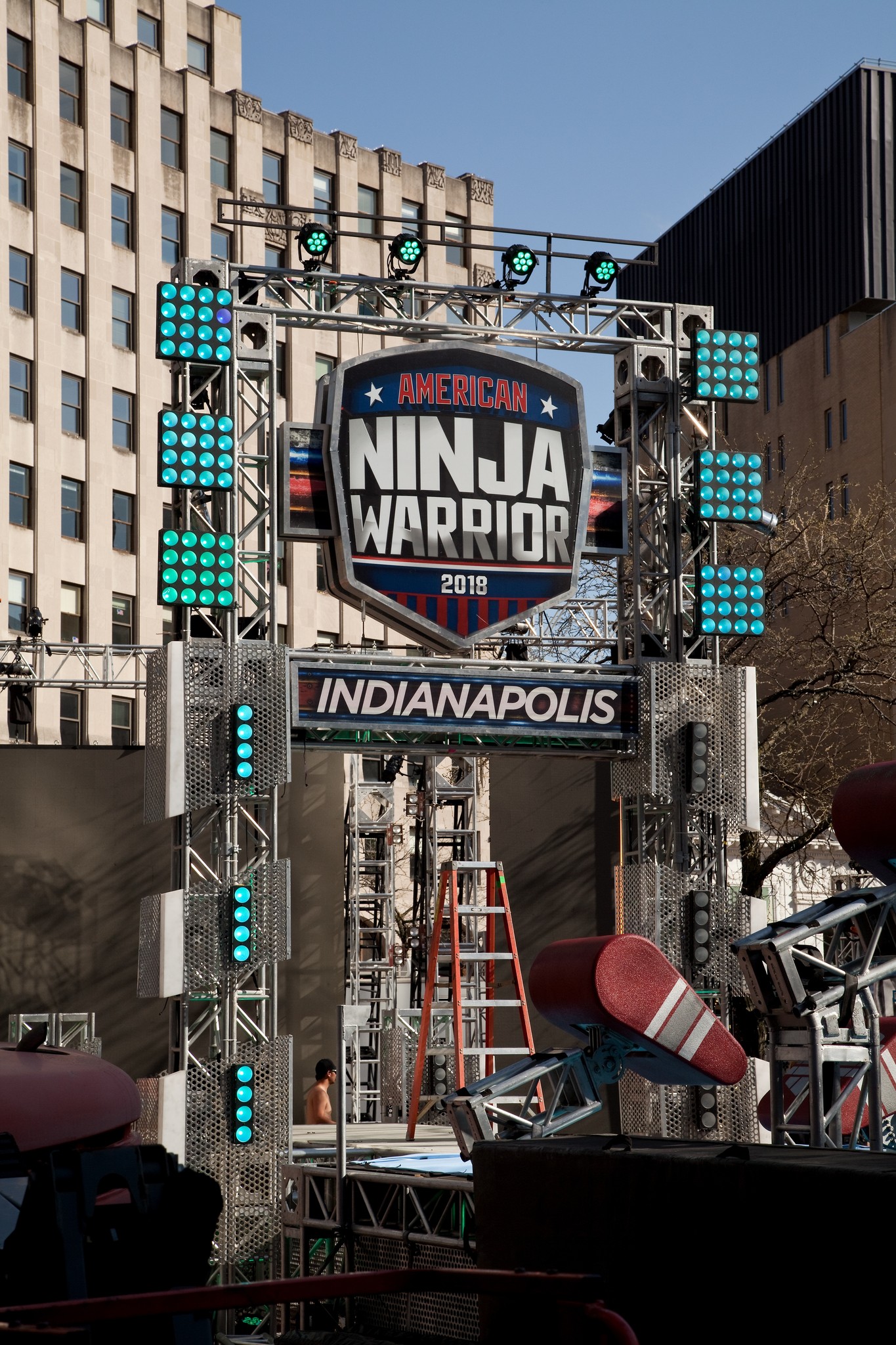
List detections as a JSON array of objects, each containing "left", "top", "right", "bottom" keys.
[{"left": 329, "top": 1070, "right": 337, "bottom": 1075}]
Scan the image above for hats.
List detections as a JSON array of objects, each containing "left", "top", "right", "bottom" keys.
[{"left": 315, "top": 1058, "right": 337, "bottom": 1075}]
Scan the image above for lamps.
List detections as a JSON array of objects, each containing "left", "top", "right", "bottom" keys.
[
  {"left": 500, "top": 243, "right": 539, "bottom": 291},
  {"left": 293, "top": 222, "right": 337, "bottom": 270},
  {"left": 377, "top": 232, "right": 426, "bottom": 279},
  {"left": 579, "top": 251, "right": 621, "bottom": 309}
]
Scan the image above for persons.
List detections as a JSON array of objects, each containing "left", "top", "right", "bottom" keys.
[{"left": 305, "top": 1059, "right": 337, "bottom": 1126}]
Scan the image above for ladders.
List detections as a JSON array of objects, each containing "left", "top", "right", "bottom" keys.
[{"left": 400, "top": 855, "right": 546, "bottom": 1145}]
